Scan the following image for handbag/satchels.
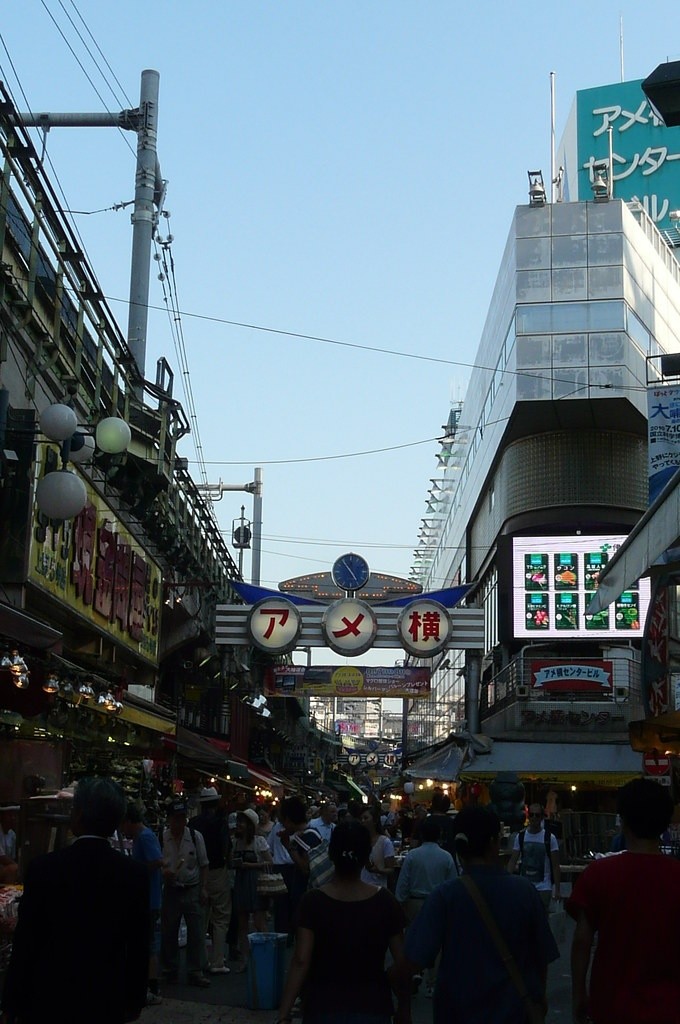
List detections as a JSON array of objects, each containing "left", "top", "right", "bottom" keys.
[
  {"left": 294, "top": 829, "right": 336, "bottom": 891},
  {"left": 526, "top": 1003, "right": 546, "bottom": 1024},
  {"left": 257, "top": 861, "right": 288, "bottom": 898}
]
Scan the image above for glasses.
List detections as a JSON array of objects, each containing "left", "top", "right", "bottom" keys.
[{"left": 528, "top": 813, "right": 541, "bottom": 818}]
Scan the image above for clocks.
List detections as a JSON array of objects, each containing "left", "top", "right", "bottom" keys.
[{"left": 333, "top": 554, "right": 370, "bottom": 590}]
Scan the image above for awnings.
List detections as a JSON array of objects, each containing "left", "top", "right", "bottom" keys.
[
  {"left": 157, "top": 726, "right": 368, "bottom": 804},
  {"left": 50, "top": 653, "right": 176, "bottom": 734},
  {"left": 398, "top": 732, "right": 674, "bottom": 787}
]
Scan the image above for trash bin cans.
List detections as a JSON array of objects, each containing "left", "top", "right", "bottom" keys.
[{"left": 247, "top": 931, "right": 288, "bottom": 1011}]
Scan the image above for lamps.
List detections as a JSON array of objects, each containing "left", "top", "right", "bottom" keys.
[
  {"left": 0, "top": 390, "right": 131, "bottom": 518},
  {"left": 233, "top": 687, "right": 271, "bottom": 718},
  {"left": 0, "top": 638, "right": 123, "bottom": 715},
  {"left": 591, "top": 162, "right": 610, "bottom": 204},
  {"left": 527, "top": 169, "right": 546, "bottom": 207}
]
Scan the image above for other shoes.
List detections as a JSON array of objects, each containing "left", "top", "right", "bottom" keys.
[
  {"left": 146, "top": 989, "right": 162, "bottom": 1005},
  {"left": 424, "top": 985, "right": 434, "bottom": 997},
  {"left": 169, "top": 968, "right": 184, "bottom": 984},
  {"left": 235, "top": 963, "right": 247, "bottom": 974},
  {"left": 188, "top": 969, "right": 212, "bottom": 987},
  {"left": 410, "top": 970, "right": 424, "bottom": 996},
  {"left": 210, "top": 965, "right": 231, "bottom": 975}
]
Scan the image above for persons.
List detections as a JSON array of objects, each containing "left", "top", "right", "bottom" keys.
[
  {"left": 564, "top": 778, "right": 680, "bottom": 1024},
  {"left": 276, "top": 820, "right": 411, "bottom": 1024},
  {"left": 157, "top": 800, "right": 210, "bottom": 986},
  {"left": 0, "top": 776, "right": 680, "bottom": 1024},
  {"left": 0, "top": 777, "right": 153, "bottom": 1024},
  {"left": 403, "top": 804, "right": 561, "bottom": 1024}
]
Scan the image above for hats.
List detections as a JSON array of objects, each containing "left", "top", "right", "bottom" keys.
[
  {"left": 167, "top": 800, "right": 187, "bottom": 816},
  {"left": 196, "top": 787, "right": 222, "bottom": 801},
  {"left": 236, "top": 805, "right": 260, "bottom": 833}
]
